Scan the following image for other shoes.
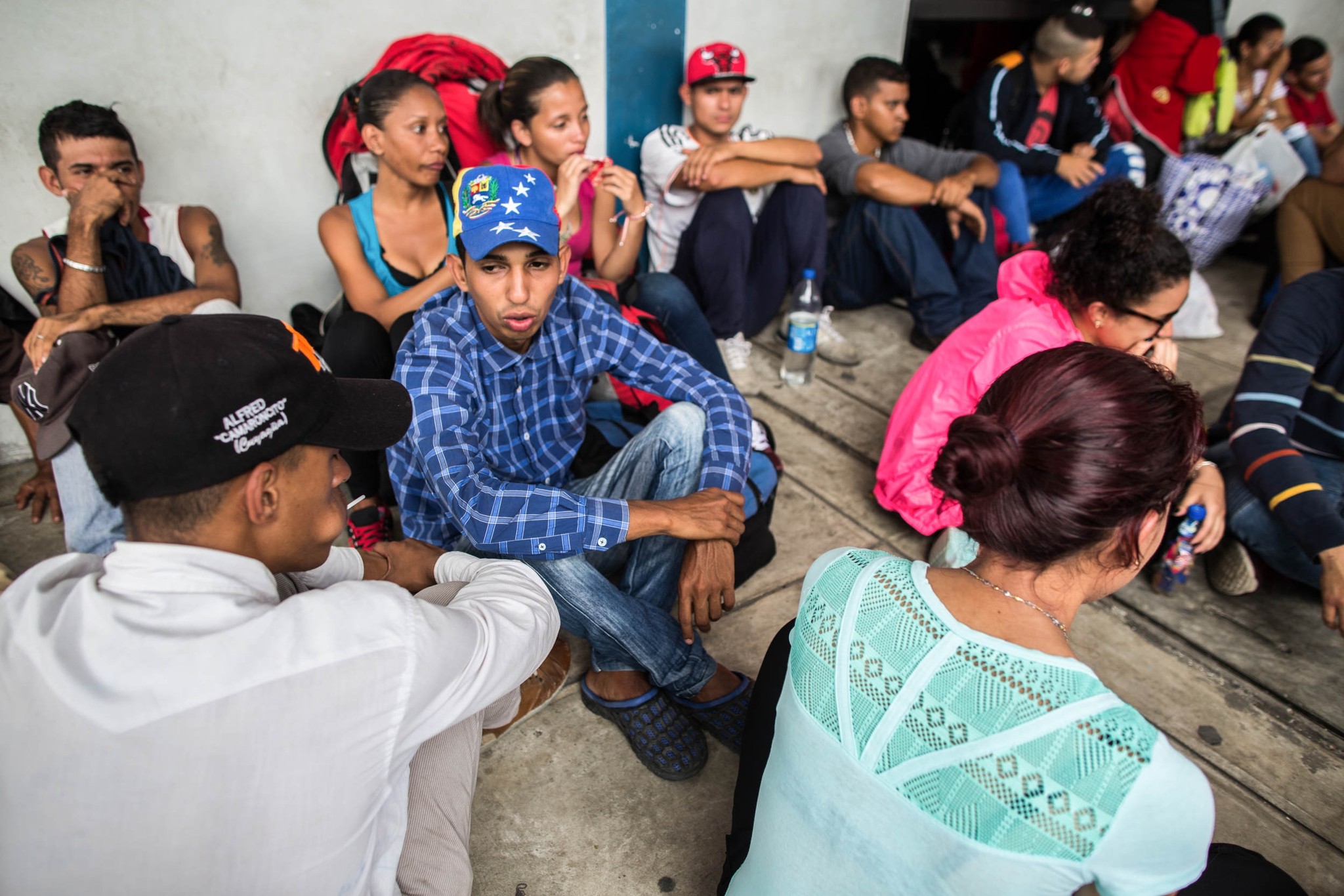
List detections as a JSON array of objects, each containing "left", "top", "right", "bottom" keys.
[
  {"left": 343, "top": 506, "right": 395, "bottom": 551},
  {"left": 750, "top": 418, "right": 787, "bottom": 482},
  {"left": 715, "top": 331, "right": 764, "bottom": 398},
  {"left": 472, "top": 635, "right": 572, "bottom": 754},
  {"left": 776, "top": 305, "right": 863, "bottom": 366},
  {"left": 1205, "top": 538, "right": 1265, "bottom": 597}
]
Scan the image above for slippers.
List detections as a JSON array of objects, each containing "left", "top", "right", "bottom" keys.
[
  {"left": 676, "top": 670, "right": 755, "bottom": 753},
  {"left": 581, "top": 674, "right": 709, "bottom": 781}
]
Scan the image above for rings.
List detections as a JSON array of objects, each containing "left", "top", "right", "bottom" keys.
[{"left": 36, "top": 334, "right": 44, "bottom": 339}]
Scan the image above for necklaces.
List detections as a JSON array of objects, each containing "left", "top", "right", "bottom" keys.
[{"left": 959, "top": 566, "right": 1071, "bottom": 641}]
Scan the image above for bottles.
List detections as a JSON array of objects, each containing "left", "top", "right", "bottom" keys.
[
  {"left": 780, "top": 269, "right": 822, "bottom": 386},
  {"left": 1148, "top": 504, "right": 1206, "bottom": 599}
]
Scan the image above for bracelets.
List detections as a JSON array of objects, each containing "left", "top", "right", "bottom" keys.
[
  {"left": 1253, "top": 96, "right": 1271, "bottom": 109},
  {"left": 62, "top": 258, "right": 106, "bottom": 273},
  {"left": 608, "top": 200, "right": 655, "bottom": 247}
]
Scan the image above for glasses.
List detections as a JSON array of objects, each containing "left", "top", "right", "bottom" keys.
[{"left": 1113, "top": 303, "right": 1180, "bottom": 340}]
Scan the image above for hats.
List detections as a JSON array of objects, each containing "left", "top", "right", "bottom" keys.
[
  {"left": 451, "top": 163, "right": 563, "bottom": 261},
  {"left": 685, "top": 43, "right": 757, "bottom": 86},
  {"left": 9, "top": 330, "right": 112, "bottom": 459},
  {"left": 64, "top": 312, "right": 413, "bottom": 500}
]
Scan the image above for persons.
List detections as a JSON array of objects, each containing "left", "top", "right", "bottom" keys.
[{"left": 0, "top": 0, "right": 1343, "bottom": 894}]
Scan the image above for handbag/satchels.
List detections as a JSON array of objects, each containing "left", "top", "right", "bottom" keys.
[
  {"left": 1155, "top": 152, "right": 1274, "bottom": 270},
  {"left": 1218, "top": 123, "right": 1307, "bottom": 225}
]
